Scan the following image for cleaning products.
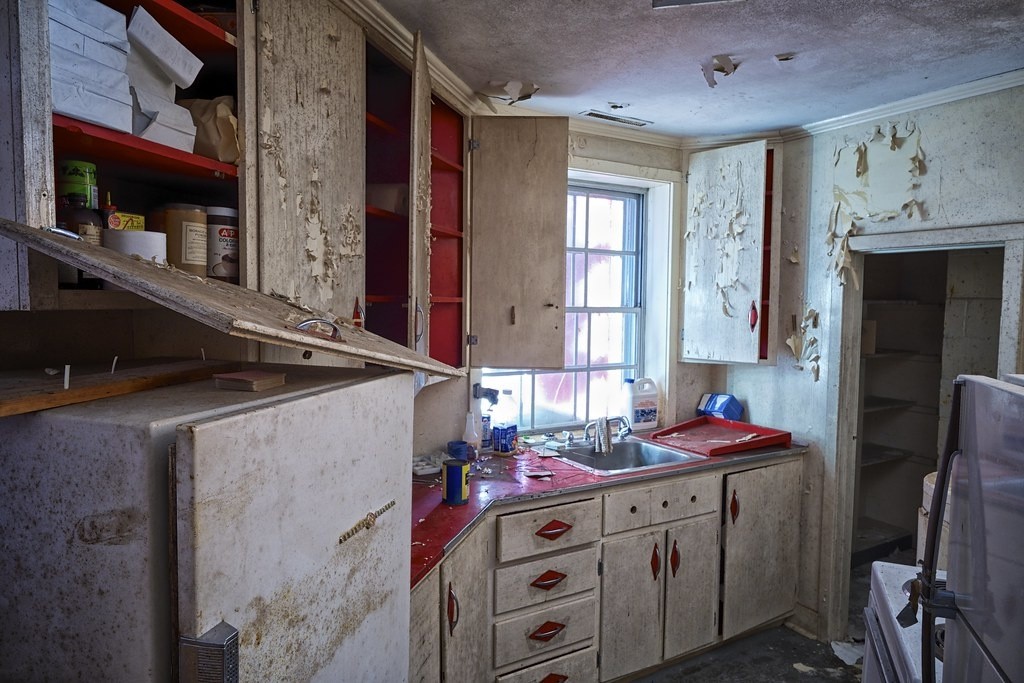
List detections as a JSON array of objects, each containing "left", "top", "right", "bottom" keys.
[
  {"left": 624, "top": 377, "right": 660, "bottom": 430},
  {"left": 471, "top": 382, "right": 500, "bottom": 454},
  {"left": 462, "top": 413, "right": 479, "bottom": 459}
]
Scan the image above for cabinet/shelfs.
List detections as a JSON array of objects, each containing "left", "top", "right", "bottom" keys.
[
  {"left": 597, "top": 469, "right": 717, "bottom": 682},
  {"left": 718, "top": 452, "right": 803, "bottom": 645},
  {"left": 0, "top": 0, "right": 569, "bottom": 371},
  {"left": 677, "top": 138, "right": 784, "bottom": 365},
  {"left": 851, "top": 300, "right": 921, "bottom": 569},
  {"left": 410, "top": 517, "right": 486, "bottom": 683},
  {"left": 485, "top": 488, "right": 598, "bottom": 683}
]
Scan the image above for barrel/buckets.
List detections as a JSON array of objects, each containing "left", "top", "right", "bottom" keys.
[
  {"left": 441, "top": 458, "right": 471, "bottom": 505},
  {"left": 922, "top": 471, "right": 951, "bottom": 522},
  {"left": 620, "top": 377, "right": 658, "bottom": 431}
]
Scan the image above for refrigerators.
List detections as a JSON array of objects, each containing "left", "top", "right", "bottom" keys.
[
  {"left": 0, "top": 360, "right": 414, "bottom": 683},
  {"left": 920, "top": 371, "right": 1023, "bottom": 683}
]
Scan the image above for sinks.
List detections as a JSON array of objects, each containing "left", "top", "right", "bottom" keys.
[{"left": 530, "top": 434, "right": 712, "bottom": 478}]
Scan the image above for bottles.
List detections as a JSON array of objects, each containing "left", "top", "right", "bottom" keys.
[
  {"left": 56, "top": 192, "right": 118, "bottom": 290},
  {"left": 462, "top": 411, "right": 478, "bottom": 460},
  {"left": 492, "top": 389, "right": 518, "bottom": 456}
]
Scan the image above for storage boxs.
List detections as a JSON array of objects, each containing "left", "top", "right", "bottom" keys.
[
  {"left": 412, "top": 455, "right": 440, "bottom": 475},
  {"left": 697, "top": 393, "right": 744, "bottom": 422}
]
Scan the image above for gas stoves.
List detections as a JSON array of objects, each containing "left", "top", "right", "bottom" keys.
[{"left": 869, "top": 561, "right": 947, "bottom": 683}]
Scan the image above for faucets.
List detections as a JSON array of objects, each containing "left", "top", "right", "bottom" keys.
[{"left": 582, "top": 414, "right": 632, "bottom": 447}]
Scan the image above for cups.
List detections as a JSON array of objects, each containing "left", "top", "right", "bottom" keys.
[{"left": 447, "top": 440, "right": 476, "bottom": 461}]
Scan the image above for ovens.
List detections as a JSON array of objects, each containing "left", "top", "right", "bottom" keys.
[{"left": 860, "top": 608, "right": 899, "bottom": 683}]
